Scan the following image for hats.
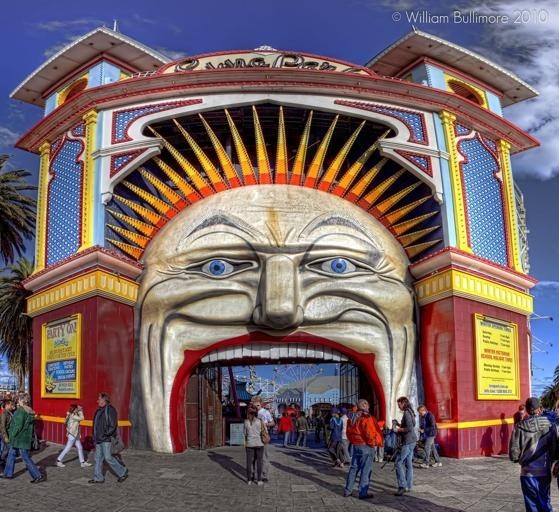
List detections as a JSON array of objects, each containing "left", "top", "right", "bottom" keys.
[
  {"left": 330, "top": 407, "right": 338, "bottom": 413},
  {"left": 69, "top": 404, "right": 78, "bottom": 413},
  {"left": 356, "top": 399, "right": 371, "bottom": 413},
  {"left": 526, "top": 397, "right": 541, "bottom": 411},
  {"left": 340, "top": 408, "right": 347, "bottom": 414}
]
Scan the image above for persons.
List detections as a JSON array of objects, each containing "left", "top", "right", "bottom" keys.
[
  {"left": 242, "top": 394, "right": 418, "bottom": 501},
  {"left": 53, "top": 404, "right": 93, "bottom": 469},
  {"left": 507, "top": 396, "right": 559, "bottom": 511},
  {"left": 0, "top": 394, "right": 45, "bottom": 483},
  {"left": 86, "top": 390, "right": 129, "bottom": 484},
  {"left": 0, "top": 398, "right": 15, "bottom": 477},
  {"left": 416, "top": 404, "right": 443, "bottom": 468}
]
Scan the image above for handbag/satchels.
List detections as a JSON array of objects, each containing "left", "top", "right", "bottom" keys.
[
  {"left": 384, "top": 430, "right": 398, "bottom": 449},
  {"left": 258, "top": 418, "right": 271, "bottom": 444},
  {"left": 32, "top": 424, "right": 39, "bottom": 450},
  {"left": 109, "top": 427, "right": 126, "bottom": 456}
]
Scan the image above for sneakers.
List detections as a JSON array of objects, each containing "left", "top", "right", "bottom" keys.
[
  {"left": 419, "top": 463, "right": 430, "bottom": 469},
  {"left": 432, "top": 462, "right": 444, "bottom": 467},
  {"left": 80, "top": 461, "right": 92, "bottom": 468},
  {"left": 56, "top": 461, "right": 66, "bottom": 467}
]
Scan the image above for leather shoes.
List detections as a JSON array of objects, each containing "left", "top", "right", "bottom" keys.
[
  {"left": 87, "top": 479, "right": 105, "bottom": 484},
  {"left": 118, "top": 475, "right": 128, "bottom": 482},
  {"left": 30, "top": 475, "right": 43, "bottom": 483},
  {"left": 344, "top": 493, "right": 352, "bottom": 497},
  {"left": 359, "top": 493, "right": 374, "bottom": 499},
  {"left": 0, "top": 475, "right": 14, "bottom": 480}
]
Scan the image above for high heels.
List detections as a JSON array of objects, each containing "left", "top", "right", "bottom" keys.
[
  {"left": 394, "top": 488, "right": 406, "bottom": 496},
  {"left": 331, "top": 461, "right": 341, "bottom": 469}
]
